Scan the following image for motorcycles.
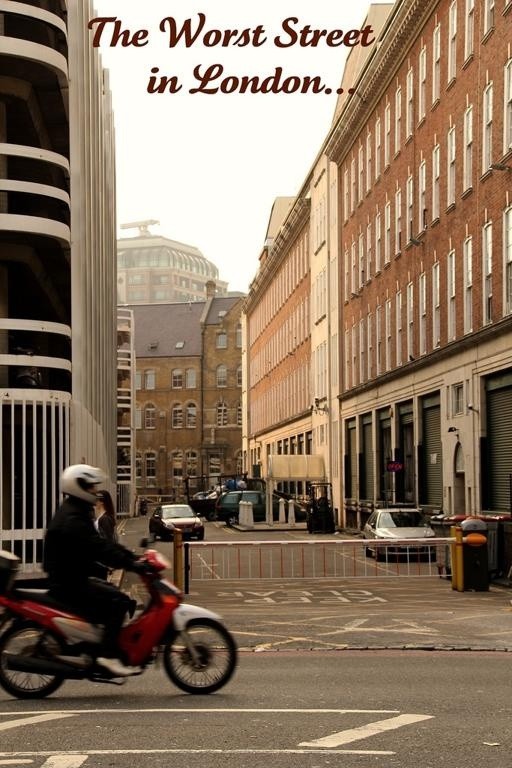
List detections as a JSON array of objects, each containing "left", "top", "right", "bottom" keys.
[{"left": 1, "top": 537, "right": 239, "bottom": 700}]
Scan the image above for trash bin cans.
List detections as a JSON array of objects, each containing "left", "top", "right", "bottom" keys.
[{"left": 429, "top": 514, "right": 512, "bottom": 592}]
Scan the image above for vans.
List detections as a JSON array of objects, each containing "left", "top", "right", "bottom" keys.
[{"left": 215, "top": 490, "right": 307, "bottom": 528}]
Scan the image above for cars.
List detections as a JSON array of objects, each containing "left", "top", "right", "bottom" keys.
[
  {"left": 192, "top": 490, "right": 226, "bottom": 500},
  {"left": 363, "top": 508, "right": 438, "bottom": 561},
  {"left": 150, "top": 504, "right": 206, "bottom": 541}
]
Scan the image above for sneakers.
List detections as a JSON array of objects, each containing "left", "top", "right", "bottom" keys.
[{"left": 97, "top": 654, "right": 131, "bottom": 678}]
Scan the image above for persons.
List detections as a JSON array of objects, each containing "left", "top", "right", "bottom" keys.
[
  {"left": 93, "top": 490, "right": 118, "bottom": 580},
  {"left": 43, "top": 464, "right": 156, "bottom": 676}
]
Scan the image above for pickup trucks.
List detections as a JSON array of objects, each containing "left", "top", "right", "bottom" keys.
[{"left": 189, "top": 477, "right": 294, "bottom": 521}]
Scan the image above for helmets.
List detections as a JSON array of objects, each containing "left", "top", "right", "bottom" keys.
[{"left": 59, "top": 464, "right": 106, "bottom": 504}]
